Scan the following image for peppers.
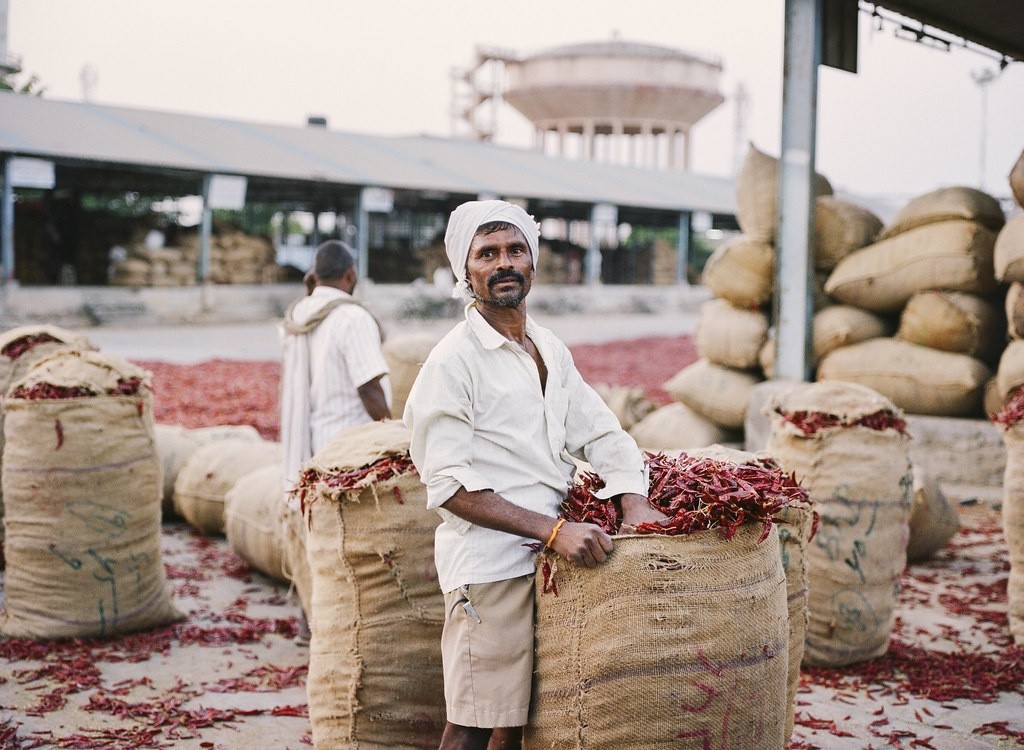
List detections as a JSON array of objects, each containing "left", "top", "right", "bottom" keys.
[{"left": 0, "top": 336, "right": 1024, "bottom": 750}]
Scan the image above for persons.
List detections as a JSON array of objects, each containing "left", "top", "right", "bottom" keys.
[
  {"left": 405, "top": 200, "right": 667, "bottom": 750},
  {"left": 283, "top": 240, "right": 392, "bottom": 456}
]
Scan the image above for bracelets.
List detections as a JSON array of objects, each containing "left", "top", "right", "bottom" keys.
[{"left": 547, "top": 520, "right": 566, "bottom": 547}]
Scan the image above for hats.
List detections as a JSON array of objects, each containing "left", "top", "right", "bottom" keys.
[{"left": 443, "top": 199, "right": 542, "bottom": 300}]
